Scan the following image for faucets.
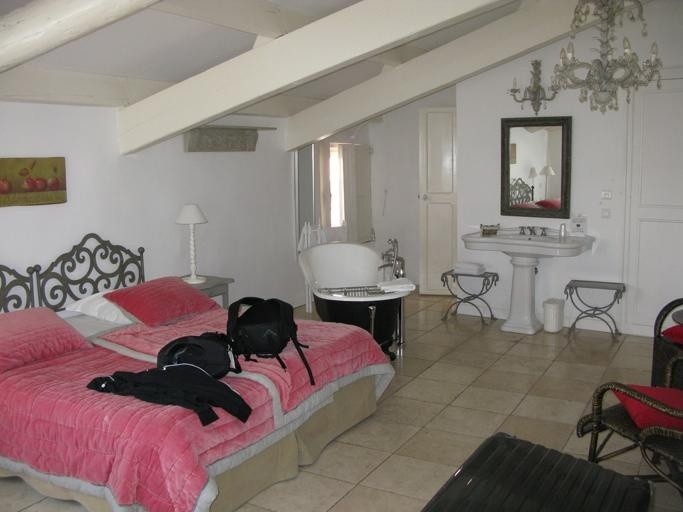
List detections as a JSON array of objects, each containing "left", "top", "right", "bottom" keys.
[
  {"left": 527, "top": 226, "right": 536, "bottom": 235},
  {"left": 378, "top": 263, "right": 393, "bottom": 271}
]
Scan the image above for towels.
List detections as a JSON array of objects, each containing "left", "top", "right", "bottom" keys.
[{"left": 375, "top": 278, "right": 416, "bottom": 293}]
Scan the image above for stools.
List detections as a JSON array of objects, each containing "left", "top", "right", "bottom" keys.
[
  {"left": 564, "top": 280, "right": 624, "bottom": 340},
  {"left": 441, "top": 269, "right": 499, "bottom": 322}
]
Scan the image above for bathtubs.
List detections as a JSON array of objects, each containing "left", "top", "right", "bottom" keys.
[{"left": 298, "top": 241, "right": 409, "bottom": 362}]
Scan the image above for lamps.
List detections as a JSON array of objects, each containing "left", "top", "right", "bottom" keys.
[
  {"left": 177, "top": 205, "right": 207, "bottom": 284},
  {"left": 540, "top": 166, "right": 556, "bottom": 199},
  {"left": 663, "top": 324, "right": 683, "bottom": 343},
  {"left": 552, "top": 0, "right": 663, "bottom": 115},
  {"left": 509, "top": 60, "right": 558, "bottom": 116},
  {"left": 349, "top": 116, "right": 383, "bottom": 140}
]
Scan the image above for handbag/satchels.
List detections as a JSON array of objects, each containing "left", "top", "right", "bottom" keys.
[{"left": 157, "top": 331, "right": 242, "bottom": 380}]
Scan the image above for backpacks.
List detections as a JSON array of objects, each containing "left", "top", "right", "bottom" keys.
[{"left": 227, "top": 296, "right": 316, "bottom": 386}]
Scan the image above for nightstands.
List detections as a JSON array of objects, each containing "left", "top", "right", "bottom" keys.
[{"left": 179, "top": 274, "right": 234, "bottom": 310}]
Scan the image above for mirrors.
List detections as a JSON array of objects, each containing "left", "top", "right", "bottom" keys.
[
  {"left": 293, "top": 139, "right": 376, "bottom": 257},
  {"left": 499, "top": 116, "right": 572, "bottom": 219}
]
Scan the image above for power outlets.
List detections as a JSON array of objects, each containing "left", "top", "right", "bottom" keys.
[{"left": 601, "top": 191, "right": 613, "bottom": 200}]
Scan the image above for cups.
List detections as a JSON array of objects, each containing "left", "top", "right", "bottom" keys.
[{"left": 559, "top": 224, "right": 567, "bottom": 238}]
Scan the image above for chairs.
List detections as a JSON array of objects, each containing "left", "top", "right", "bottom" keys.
[
  {"left": 651, "top": 297, "right": 683, "bottom": 389},
  {"left": 577, "top": 355, "right": 683, "bottom": 496},
  {"left": 417, "top": 430, "right": 655, "bottom": 512}
]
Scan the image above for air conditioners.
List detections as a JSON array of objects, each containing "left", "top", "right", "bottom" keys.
[{"left": 184, "top": 127, "right": 258, "bottom": 152}]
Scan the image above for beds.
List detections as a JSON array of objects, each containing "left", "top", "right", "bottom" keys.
[
  {"left": 0, "top": 266, "right": 299, "bottom": 512},
  {"left": 506, "top": 178, "right": 561, "bottom": 210},
  {"left": 35, "top": 232, "right": 396, "bottom": 467}
]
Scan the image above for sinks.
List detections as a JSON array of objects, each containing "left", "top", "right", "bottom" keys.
[{"left": 462, "top": 230, "right": 595, "bottom": 259}]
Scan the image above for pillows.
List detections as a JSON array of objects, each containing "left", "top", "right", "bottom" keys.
[
  {"left": 511, "top": 204, "right": 536, "bottom": 209},
  {"left": 0, "top": 307, "right": 93, "bottom": 372},
  {"left": 611, "top": 382, "right": 683, "bottom": 429},
  {"left": 103, "top": 276, "right": 221, "bottom": 327},
  {"left": 535, "top": 199, "right": 561, "bottom": 209},
  {"left": 66, "top": 288, "right": 138, "bottom": 324}
]
